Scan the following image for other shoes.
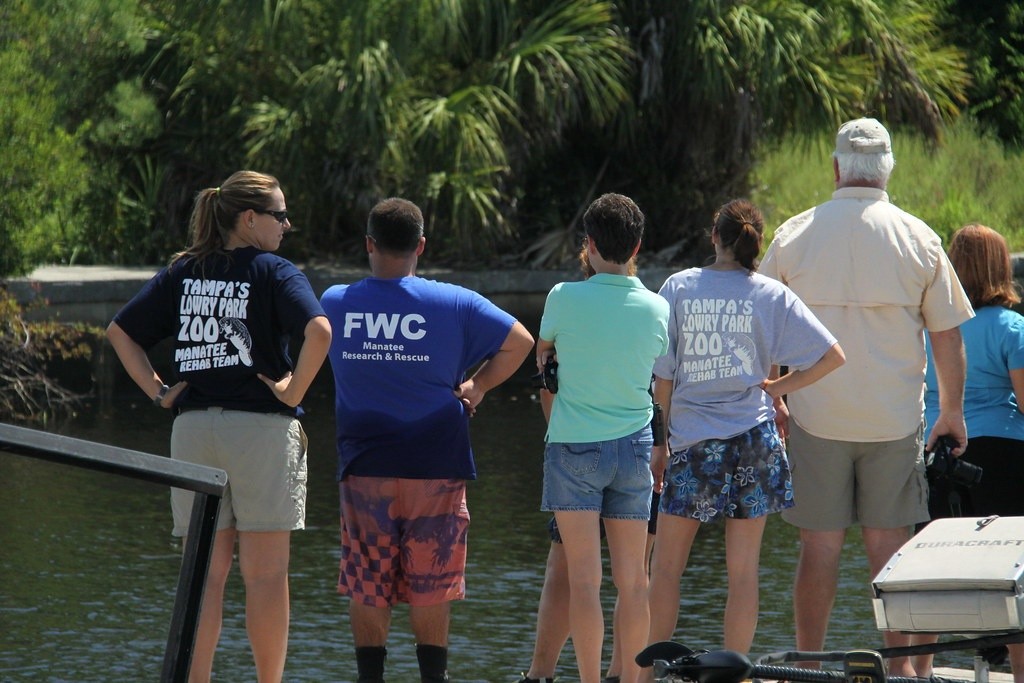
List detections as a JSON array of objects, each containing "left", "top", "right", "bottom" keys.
[{"left": 512, "top": 671, "right": 560, "bottom": 683}]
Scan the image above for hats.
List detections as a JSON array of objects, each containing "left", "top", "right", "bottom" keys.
[{"left": 835, "top": 116, "right": 892, "bottom": 154}]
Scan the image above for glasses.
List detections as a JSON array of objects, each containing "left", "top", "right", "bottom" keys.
[{"left": 245, "top": 204, "right": 288, "bottom": 223}]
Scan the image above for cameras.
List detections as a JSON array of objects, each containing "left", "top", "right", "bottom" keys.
[{"left": 925, "top": 436, "right": 983, "bottom": 489}]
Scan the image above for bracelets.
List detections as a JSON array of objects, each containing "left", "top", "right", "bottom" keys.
[{"left": 155, "top": 385, "right": 168, "bottom": 406}]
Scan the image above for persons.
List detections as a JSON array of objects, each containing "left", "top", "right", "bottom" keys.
[
  {"left": 638, "top": 198, "right": 846, "bottom": 683},
  {"left": 912, "top": 225, "right": 1024, "bottom": 681},
  {"left": 320, "top": 198, "right": 534, "bottom": 683},
  {"left": 516, "top": 194, "right": 669, "bottom": 683},
  {"left": 106, "top": 171, "right": 332, "bottom": 683},
  {"left": 760, "top": 119, "right": 974, "bottom": 678}
]
